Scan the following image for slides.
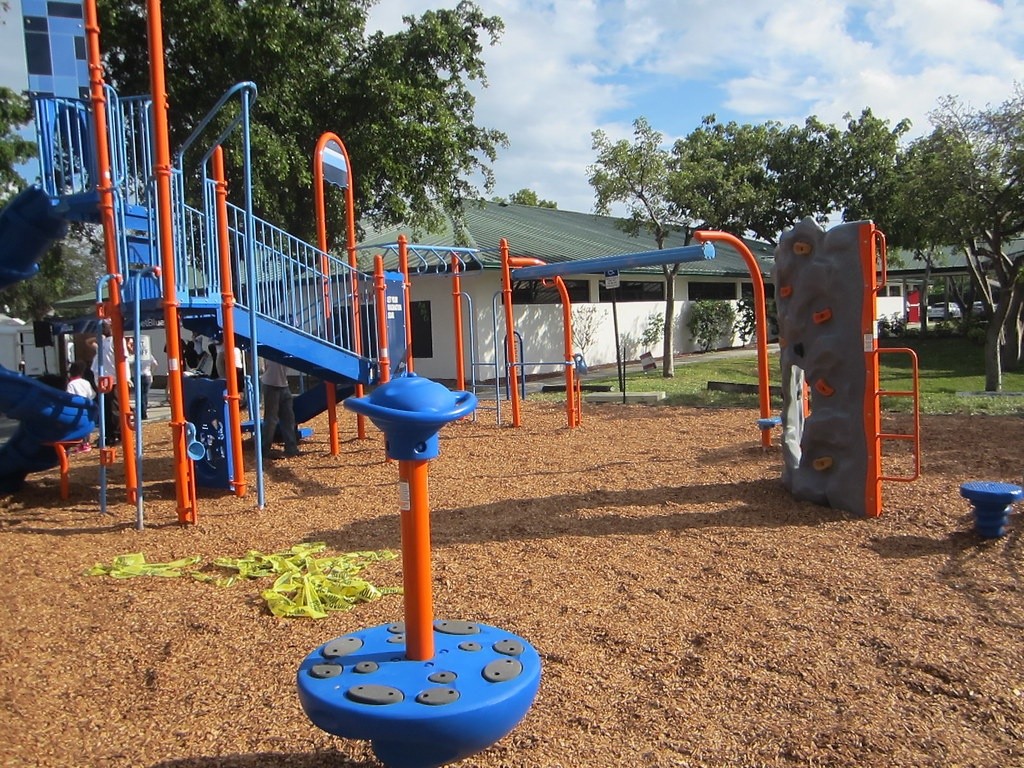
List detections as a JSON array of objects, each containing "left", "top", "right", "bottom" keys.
[{"left": 1, "top": 184, "right": 101, "bottom": 485}]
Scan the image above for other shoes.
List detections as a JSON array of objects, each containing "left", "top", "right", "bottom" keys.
[
  {"left": 261, "top": 449, "right": 283, "bottom": 459},
  {"left": 80, "top": 442, "right": 92, "bottom": 452},
  {"left": 97, "top": 431, "right": 122, "bottom": 447},
  {"left": 284, "top": 450, "right": 307, "bottom": 457},
  {"left": 142, "top": 415, "right": 147, "bottom": 419}
]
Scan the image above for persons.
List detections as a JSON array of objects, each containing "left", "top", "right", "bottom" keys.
[
  {"left": 65, "top": 361, "right": 96, "bottom": 453},
  {"left": 163, "top": 332, "right": 305, "bottom": 459},
  {"left": 84, "top": 324, "right": 159, "bottom": 445}
]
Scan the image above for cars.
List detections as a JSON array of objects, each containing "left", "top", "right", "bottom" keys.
[
  {"left": 928, "top": 301, "right": 961, "bottom": 322},
  {"left": 971, "top": 301, "right": 998, "bottom": 315}
]
